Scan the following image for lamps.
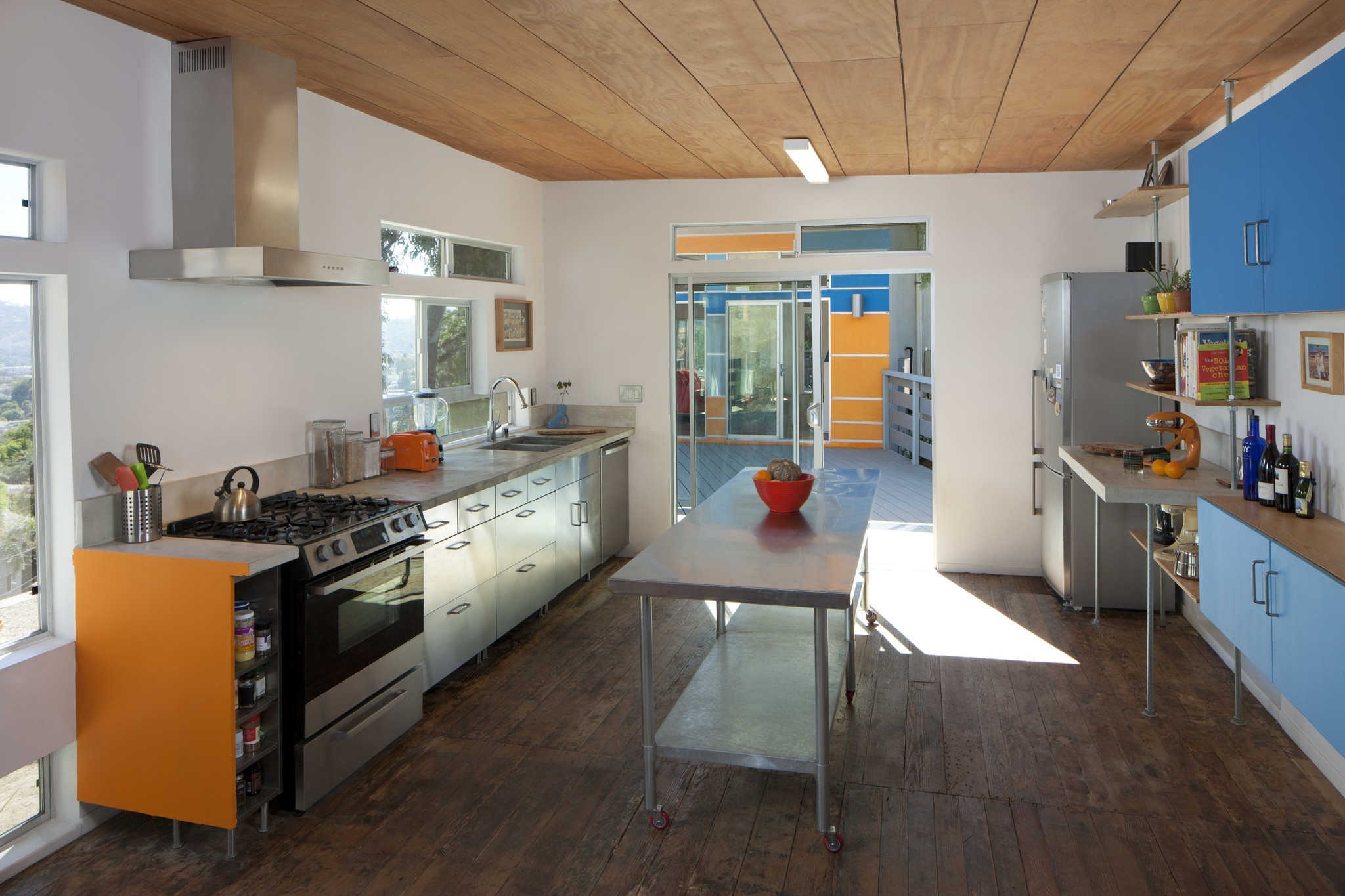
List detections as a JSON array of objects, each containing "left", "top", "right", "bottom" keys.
[
  {"left": 783, "top": 139, "right": 829, "bottom": 184},
  {"left": 852, "top": 294, "right": 863, "bottom": 317}
]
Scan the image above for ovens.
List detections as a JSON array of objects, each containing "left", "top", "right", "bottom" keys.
[{"left": 280, "top": 534, "right": 425, "bottom": 812}]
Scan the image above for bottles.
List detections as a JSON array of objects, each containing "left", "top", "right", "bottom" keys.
[
  {"left": 1295, "top": 461, "right": 1316, "bottom": 519},
  {"left": 1274, "top": 433, "right": 1299, "bottom": 513},
  {"left": 1258, "top": 424, "right": 1280, "bottom": 507},
  {"left": 1241, "top": 415, "right": 1266, "bottom": 501},
  {"left": 233, "top": 599, "right": 272, "bottom": 803},
  {"left": 312, "top": 419, "right": 397, "bottom": 490}
]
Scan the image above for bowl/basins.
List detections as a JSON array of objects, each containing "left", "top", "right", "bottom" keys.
[
  {"left": 1102, "top": 199, "right": 1118, "bottom": 208},
  {"left": 752, "top": 473, "right": 816, "bottom": 512},
  {"left": 1139, "top": 359, "right": 1175, "bottom": 384}
]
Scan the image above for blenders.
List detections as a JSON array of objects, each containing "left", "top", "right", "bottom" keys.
[
  {"left": 1143, "top": 411, "right": 1201, "bottom": 468},
  {"left": 411, "top": 388, "right": 449, "bottom": 463}
]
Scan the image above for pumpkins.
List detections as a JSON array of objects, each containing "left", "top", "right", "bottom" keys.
[{"left": 767, "top": 458, "right": 802, "bottom": 482}]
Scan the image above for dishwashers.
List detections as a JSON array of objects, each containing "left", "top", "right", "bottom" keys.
[{"left": 599, "top": 437, "right": 631, "bottom": 564}]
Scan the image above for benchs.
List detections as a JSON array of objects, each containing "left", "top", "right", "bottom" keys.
[{"left": 676, "top": 369, "right": 705, "bottom": 420}]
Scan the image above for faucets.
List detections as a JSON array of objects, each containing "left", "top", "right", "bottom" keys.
[{"left": 487, "top": 377, "right": 529, "bottom": 442}]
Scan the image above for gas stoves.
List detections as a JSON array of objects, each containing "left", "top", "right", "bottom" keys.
[{"left": 161, "top": 490, "right": 429, "bottom": 577}]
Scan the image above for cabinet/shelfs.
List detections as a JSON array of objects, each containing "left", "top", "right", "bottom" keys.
[
  {"left": 73, "top": 534, "right": 300, "bottom": 861},
  {"left": 555, "top": 471, "right": 601, "bottom": 597},
  {"left": 421, "top": 486, "right": 497, "bottom": 697},
  {"left": 1187, "top": 49, "right": 1345, "bottom": 314},
  {"left": 1095, "top": 141, "right": 1281, "bottom": 604},
  {"left": 1197, "top": 494, "right": 1345, "bottom": 759},
  {"left": 495, "top": 463, "right": 556, "bottom": 640}
]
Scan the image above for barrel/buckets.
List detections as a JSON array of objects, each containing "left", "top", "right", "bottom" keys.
[{"left": 1173, "top": 530, "right": 1198, "bottom": 580}]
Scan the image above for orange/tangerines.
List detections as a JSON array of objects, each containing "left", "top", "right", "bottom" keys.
[
  {"left": 1151, "top": 460, "right": 1168, "bottom": 476},
  {"left": 1165, "top": 461, "right": 1186, "bottom": 479},
  {"left": 755, "top": 469, "right": 781, "bottom": 482}
]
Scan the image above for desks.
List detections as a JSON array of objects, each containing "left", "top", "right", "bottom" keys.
[{"left": 1059, "top": 445, "right": 1248, "bottom": 726}]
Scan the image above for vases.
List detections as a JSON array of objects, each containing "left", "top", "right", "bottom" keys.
[{"left": 546, "top": 405, "right": 569, "bottom": 428}]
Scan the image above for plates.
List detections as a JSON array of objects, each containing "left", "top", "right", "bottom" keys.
[{"left": 1146, "top": 382, "right": 1175, "bottom": 390}]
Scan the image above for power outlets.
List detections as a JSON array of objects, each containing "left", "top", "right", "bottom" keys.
[
  {"left": 370, "top": 413, "right": 380, "bottom": 438},
  {"left": 531, "top": 388, "right": 536, "bottom": 406}
]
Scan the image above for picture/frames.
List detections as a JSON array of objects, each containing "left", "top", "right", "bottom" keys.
[
  {"left": 495, "top": 298, "right": 533, "bottom": 351},
  {"left": 1142, "top": 159, "right": 1154, "bottom": 187},
  {"left": 1300, "top": 331, "right": 1345, "bottom": 395}
]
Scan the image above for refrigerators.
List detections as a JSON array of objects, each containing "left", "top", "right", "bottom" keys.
[{"left": 1029, "top": 271, "right": 1175, "bottom": 615}]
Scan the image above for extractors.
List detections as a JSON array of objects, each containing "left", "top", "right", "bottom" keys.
[{"left": 129, "top": 34, "right": 390, "bottom": 286}]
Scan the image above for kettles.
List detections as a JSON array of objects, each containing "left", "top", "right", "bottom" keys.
[{"left": 212, "top": 465, "right": 264, "bottom": 523}]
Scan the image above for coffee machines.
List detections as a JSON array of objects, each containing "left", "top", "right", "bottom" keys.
[{"left": 1145, "top": 502, "right": 1187, "bottom": 546}]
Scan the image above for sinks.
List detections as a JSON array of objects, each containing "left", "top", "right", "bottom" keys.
[
  {"left": 489, "top": 444, "right": 561, "bottom": 452},
  {"left": 507, "top": 435, "right": 586, "bottom": 446}
]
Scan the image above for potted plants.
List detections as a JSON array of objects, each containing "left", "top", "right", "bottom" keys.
[{"left": 1142, "top": 257, "right": 1191, "bottom": 313}]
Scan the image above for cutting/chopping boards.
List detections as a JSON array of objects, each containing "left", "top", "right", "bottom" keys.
[{"left": 1081, "top": 442, "right": 1149, "bottom": 455}]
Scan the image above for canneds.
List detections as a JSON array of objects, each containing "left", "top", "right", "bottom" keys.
[
  {"left": 234, "top": 609, "right": 272, "bottom": 662},
  {"left": 234, "top": 712, "right": 262, "bottom": 808},
  {"left": 235, "top": 666, "right": 266, "bottom": 710}
]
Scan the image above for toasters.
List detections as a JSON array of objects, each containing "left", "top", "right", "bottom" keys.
[{"left": 384, "top": 431, "right": 439, "bottom": 472}]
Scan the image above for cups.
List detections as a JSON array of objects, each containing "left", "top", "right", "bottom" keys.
[
  {"left": 1123, "top": 449, "right": 1144, "bottom": 474},
  {"left": 1151, "top": 445, "right": 1171, "bottom": 463}
]
